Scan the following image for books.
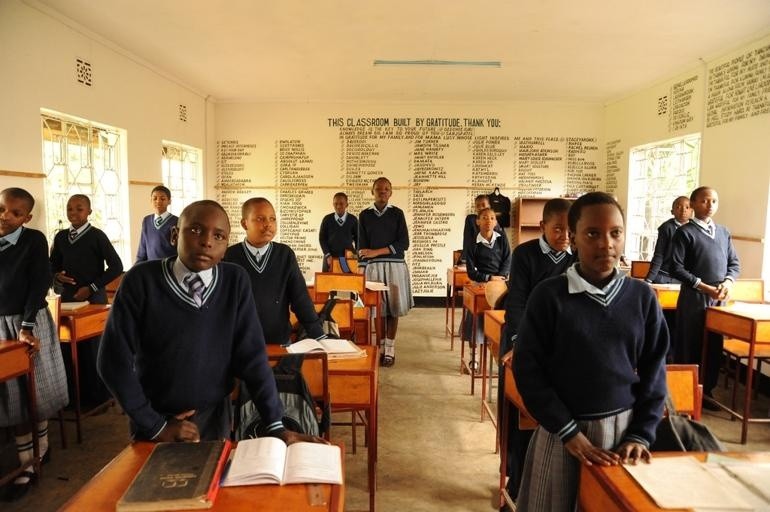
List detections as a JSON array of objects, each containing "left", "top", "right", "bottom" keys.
[
  {"left": 60, "top": 301, "right": 90, "bottom": 311},
  {"left": 285, "top": 338, "right": 367, "bottom": 359},
  {"left": 219, "top": 437, "right": 343, "bottom": 487},
  {"left": 115, "top": 440, "right": 231, "bottom": 511}
]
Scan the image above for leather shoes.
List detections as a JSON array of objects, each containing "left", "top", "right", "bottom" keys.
[
  {"left": 7, "top": 470, "right": 34, "bottom": 501},
  {"left": 380, "top": 353, "right": 395, "bottom": 366}
]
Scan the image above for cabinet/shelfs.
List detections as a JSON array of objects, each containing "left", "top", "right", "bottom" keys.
[{"left": 513, "top": 197, "right": 580, "bottom": 248}]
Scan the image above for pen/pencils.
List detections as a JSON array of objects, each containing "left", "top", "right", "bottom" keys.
[{"left": 219, "top": 459, "right": 231, "bottom": 488}]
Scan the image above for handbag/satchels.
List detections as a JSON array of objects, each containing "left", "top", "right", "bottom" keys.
[{"left": 484, "top": 188, "right": 511, "bottom": 229}]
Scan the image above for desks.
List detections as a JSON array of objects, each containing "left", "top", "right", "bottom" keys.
[
  {"left": 445, "top": 263, "right": 770, "bottom": 510},
  {"left": 0, "top": 284, "right": 384, "bottom": 511}
]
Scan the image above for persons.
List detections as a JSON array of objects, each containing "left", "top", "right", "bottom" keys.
[
  {"left": 221, "top": 197, "right": 330, "bottom": 346},
  {"left": 357, "top": 177, "right": 415, "bottom": 368},
  {"left": 133, "top": 185, "right": 179, "bottom": 264},
  {"left": 47, "top": 194, "right": 123, "bottom": 413},
  {"left": 95, "top": 200, "right": 331, "bottom": 448},
  {"left": 318, "top": 192, "right": 358, "bottom": 273},
  {"left": 497, "top": 198, "right": 574, "bottom": 504},
  {"left": 670, "top": 186, "right": 741, "bottom": 412},
  {"left": 0, "top": 186, "right": 69, "bottom": 496},
  {"left": 512, "top": 191, "right": 670, "bottom": 512},
  {"left": 462, "top": 195, "right": 511, "bottom": 372},
  {"left": 645, "top": 196, "right": 693, "bottom": 286}
]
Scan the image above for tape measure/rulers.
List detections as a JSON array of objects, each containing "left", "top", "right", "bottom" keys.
[{"left": 308, "top": 483, "right": 327, "bottom": 506}]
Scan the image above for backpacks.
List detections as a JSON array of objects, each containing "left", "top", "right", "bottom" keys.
[
  {"left": 293, "top": 300, "right": 341, "bottom": 341},
  {"left": 231, "top": 353, "right": 330, "bottom": 442},
  {"left": 650, "top": 395, "right": 725, "bottom": 452}
]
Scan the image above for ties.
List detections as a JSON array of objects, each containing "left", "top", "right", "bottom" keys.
[
  {"left": 155, "top": 217, "right": 162, "bottom": 226},
  {"left": 185, "top": 274, "right": 204, "bottom": 307},
  {"left": 71, "top": 230, "right": 78, "bottom": 240}
]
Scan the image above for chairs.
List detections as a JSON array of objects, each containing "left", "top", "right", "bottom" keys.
[
  {"left": 315, "top": 274, "right": 366, "bottom": 294},
  {"left": 331, "top": 258, "right": 360, "bottom": 274},
  {"left": 453, "top": 250, "right": 462, "bottom": 266},
  {"left": 106, "top": 271, "right": 126, "bottom": 303}
]
